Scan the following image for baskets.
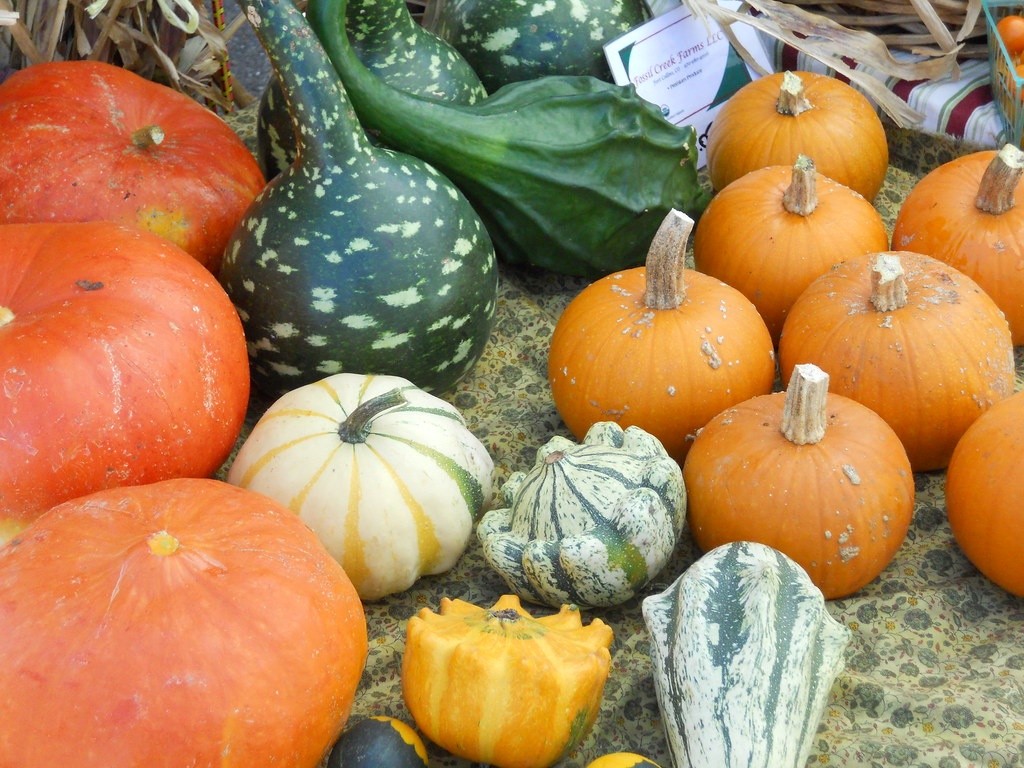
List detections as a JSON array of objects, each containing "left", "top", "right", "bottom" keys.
[{"left": 981, "top": 0, "right": 1024, "bottom": 149}]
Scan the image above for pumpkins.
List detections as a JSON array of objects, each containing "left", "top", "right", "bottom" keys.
[{"left": 0, "top": 0, "right": 1024, "bottom": 768}]
